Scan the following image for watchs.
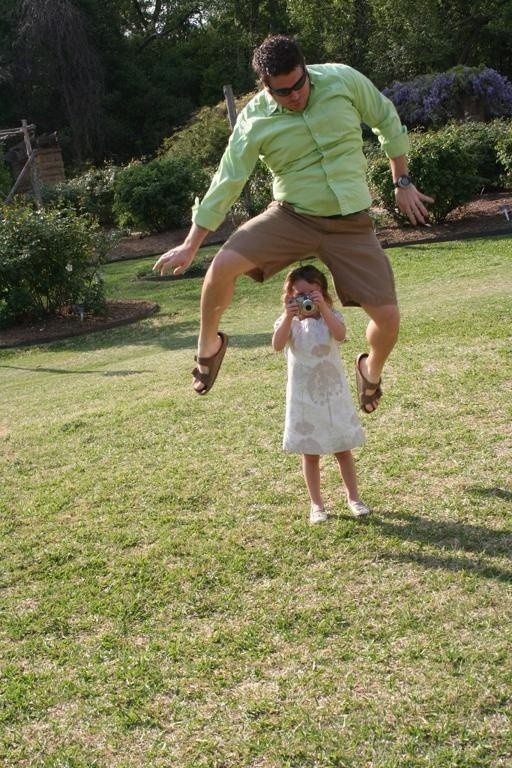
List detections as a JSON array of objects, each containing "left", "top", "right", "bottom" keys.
[{"left": 393, "top": 175, "right": 411, "bottom": 189}]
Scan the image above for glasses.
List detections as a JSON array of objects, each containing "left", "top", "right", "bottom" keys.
[{"left": 268, "top": 68, "right": 306, "bottom": 97}]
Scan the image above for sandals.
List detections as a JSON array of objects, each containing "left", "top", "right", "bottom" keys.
[
  {"left": 191, "top": 331, "right": 229, "bottom": 395},
  {"left": 355, "top": 352, "right": 383, "bottom": 414},
  {"left": 309, "top": 506, "right": 327, "bottom": 523},
  {"left": 349, "top": 501, "right": 368, "bottom": 516}
]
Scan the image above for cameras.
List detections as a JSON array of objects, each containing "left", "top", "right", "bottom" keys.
[{"left": 290, "top": 295, "right": 318, "bottom": 314}]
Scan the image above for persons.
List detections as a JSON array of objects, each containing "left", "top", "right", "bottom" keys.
[
  {"left": 152, "top": 36, "right": 435, "bottom": 414},
  {"left": 272, "top": 265, "right": 370, "bottom": 523}
]
